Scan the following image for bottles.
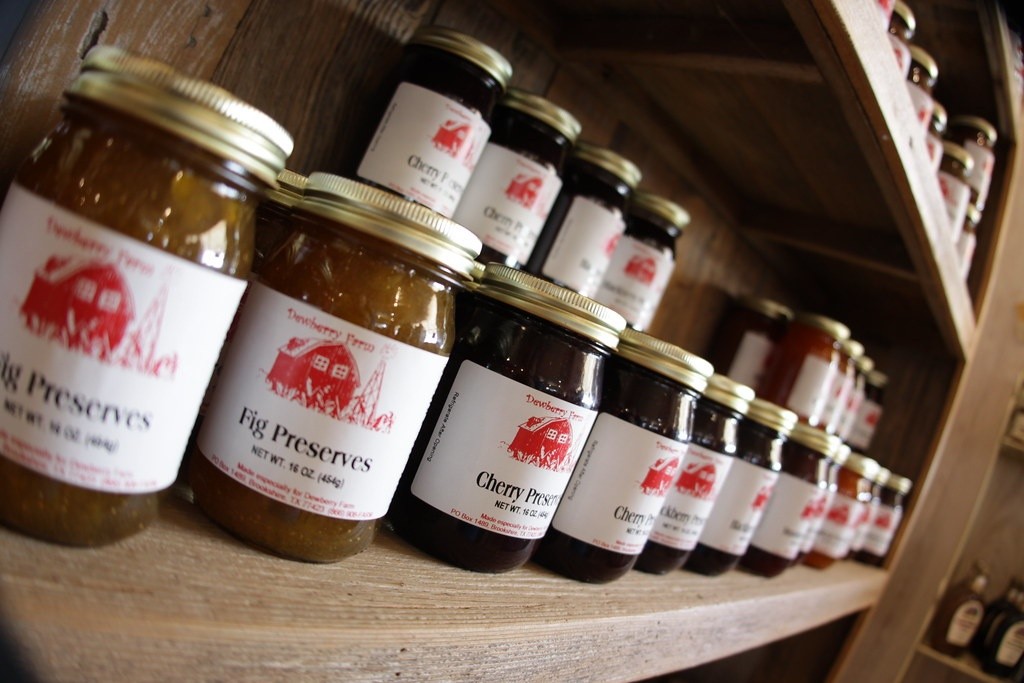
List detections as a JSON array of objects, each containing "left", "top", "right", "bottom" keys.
[
  {"left": 930, "top": 561, "right": 1024, "bottom": 683},
  {"left": 0, "top": 6, "right": 997, "bottom": 585}
]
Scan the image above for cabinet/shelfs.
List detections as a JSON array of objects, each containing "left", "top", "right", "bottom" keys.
[{"left": 0, "top": 0, "right": 1024, "bottom": 683}]
